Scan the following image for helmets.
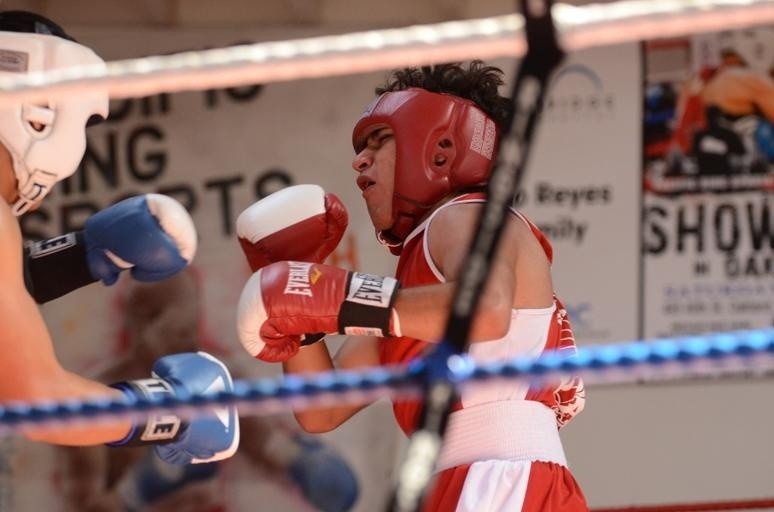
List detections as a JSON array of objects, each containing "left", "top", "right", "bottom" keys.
[
  {"left": 0, "top": 11, "right": 109, "bottom": 201},
  {"left": 351, "top": 87, "right": 499, "bottom": 253}
]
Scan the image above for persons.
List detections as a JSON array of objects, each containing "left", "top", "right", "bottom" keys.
[
  {"left": 0, "top": 6, "right": 244, "bottom": 512},
  {"left": 639, "top": 48, "right": 774, "bottom": 197},
  {"left": 232, "top": 58, "right": 591, "bottom": 512},
  {"left": 46, "top": 257, "right": 362, "bottom": 512}
]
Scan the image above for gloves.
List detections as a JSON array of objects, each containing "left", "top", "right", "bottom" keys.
[
  {"left": 81, "top": 193, "right": 198, "bottom": 286},
  {"left": 233, "top": 183, "right": 397, "bottom": 363},
  {"left": 104, "top": 350, "right": 240, "bottom": 467}
]
